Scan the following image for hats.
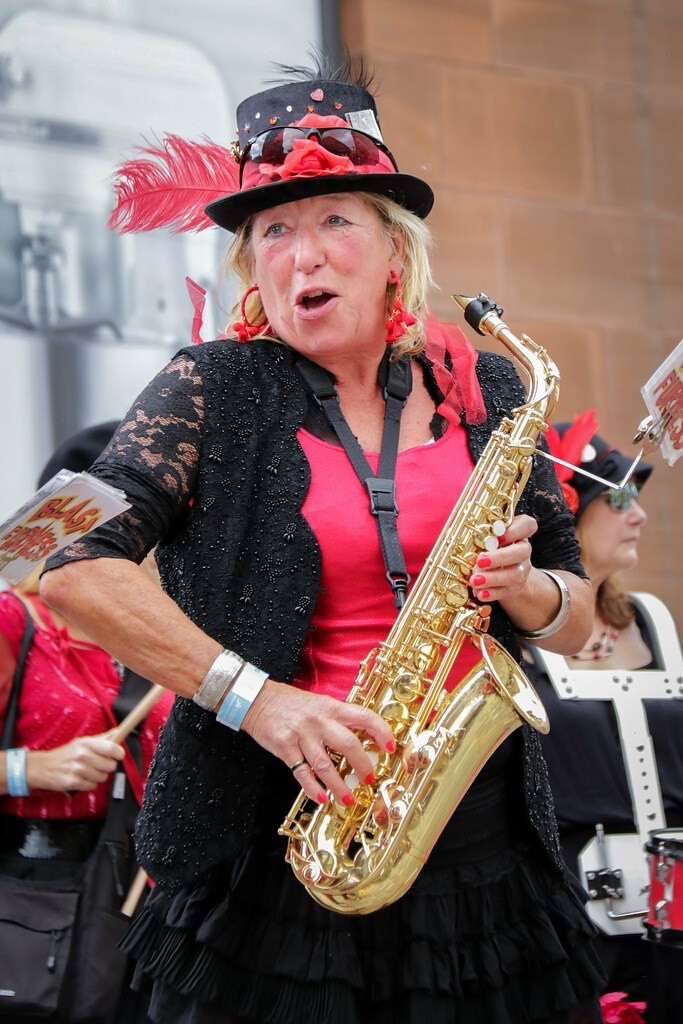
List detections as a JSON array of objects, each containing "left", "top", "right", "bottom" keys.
[
  {"left": 538, "top": 407, "right": 654, "bottom": 526},
  {"left": 35, "top": 419, "right": 120, "bottom": 488},
  {"left": 106, "top": 45, "right": 435, "bottom": 237}
]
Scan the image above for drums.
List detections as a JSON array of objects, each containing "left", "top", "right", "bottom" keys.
[{"left": 639, "top": 827, "right": 683, "bottom": 950}]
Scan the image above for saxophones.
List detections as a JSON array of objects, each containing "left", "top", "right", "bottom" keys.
[{"left": 274, "top": 290, "right": 563, "bottom": 917}]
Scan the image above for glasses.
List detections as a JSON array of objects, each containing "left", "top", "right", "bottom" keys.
[
  {"left": 239, "top": 125, "right": 399, "bottom": 191},
  {"left": 599, "top": 478, "right": 641, "bottom": 514}
]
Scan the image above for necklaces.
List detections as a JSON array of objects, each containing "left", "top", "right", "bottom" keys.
[{"left": 571, "top": 628, "right": 619, "bottom": 660}]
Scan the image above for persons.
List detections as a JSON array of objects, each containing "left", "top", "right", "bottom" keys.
[
  {"left": 496, "top": 409, "right": 683, "bottom": 1024},
  {"left": 0, "top": 422, "right": 230, "bottom": 1024},
  {"left": 39, "top": 48, "right": 609, "bottom": 1024}
]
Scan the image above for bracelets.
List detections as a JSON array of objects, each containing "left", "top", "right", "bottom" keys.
[
  {"left": 507, "top": 568, "right": 571, "bottom": 640},
  {"left": 193, "top": 647, "right": 246, "bottom": 712},
  {"left": 215, "top": 661, "right": 270, "bottom": 732},
  {"left": 5, "top": 749, "right": 30, "bottom": 798}
]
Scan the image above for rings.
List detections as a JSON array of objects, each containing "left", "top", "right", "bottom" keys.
[{"left": 289, "top": 759, "right": 307, "bottom": 771}]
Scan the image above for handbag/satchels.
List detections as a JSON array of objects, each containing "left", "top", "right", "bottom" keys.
[{"left": 0, "top": 877, "right": 84, "bottom": 1012}]
